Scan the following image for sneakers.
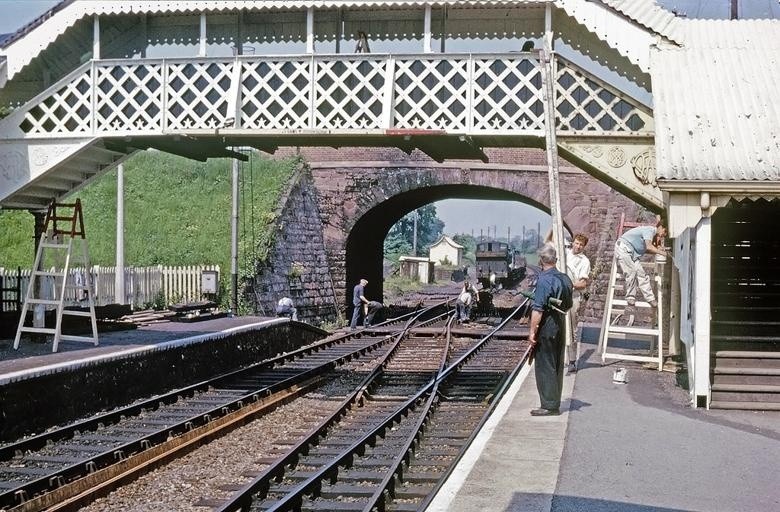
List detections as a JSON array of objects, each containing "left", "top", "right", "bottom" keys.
[
  {"left": 530, "top": 406, "right": 562, "bottom": 417},
  {"left": 627, "top": 298, "right": 636, "bottom": 306},
  {"left": 568, "top": 363, "right": 578, "bottom": 373},
  {"left": 649, "top": 300, "right": 658, "bottom": 308}
]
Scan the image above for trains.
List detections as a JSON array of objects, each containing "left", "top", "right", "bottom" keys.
[{"left": 476, "top": 241, "right": 527, "bottom": 289}]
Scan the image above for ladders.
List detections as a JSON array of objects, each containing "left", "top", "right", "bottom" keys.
[
  {"left": 597, "top": 211, "right": 663, "bottom": 372},
  {"left": 13, "top": 197, "right": 99, "bottom": 353}
]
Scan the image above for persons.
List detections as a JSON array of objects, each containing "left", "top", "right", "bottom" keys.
[
  {"left": 522, "top": 41, "right": 534, "bottom": 51},
  {"left": 529, "top": 226, "right": 591, "bottom": 415},
  {"left": 350, "top": 279, "right": 384, "bottom": 331},
  {"left": 614, "top": 222, "right": 672, "bottom": 307},
  {"left": 277, "top": 297, "right": 298, "bottom": 319},
  {"left": 490, "top": 272, "right": 498, "bottom": 295},
  {"left": 452, "top": 281, "right": 479, "bottom": 323}
]
